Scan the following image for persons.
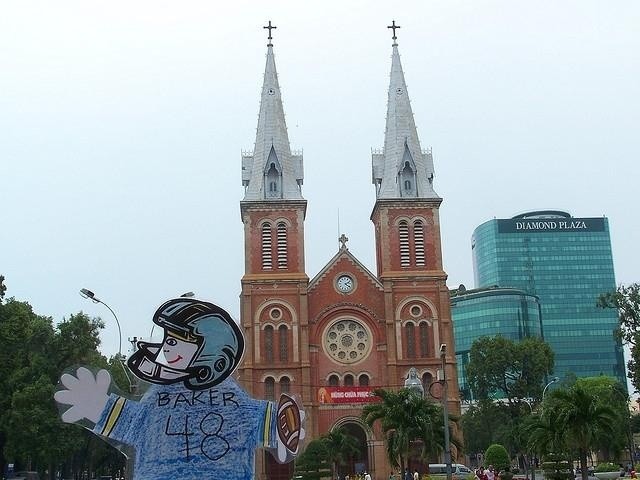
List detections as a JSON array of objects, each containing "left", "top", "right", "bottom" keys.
[
  {"left": 574, "top": 462, "right": 595, "bottom": 477},
  {"left": 477, "top": 467, "right": 488, "bottom": 480},
  {"left": 338, "top": 466, "right": 420, "bottom": 480},
  {"left": 627, "top": 462, "right": 633, "bottom": 474},
  {"left": 405, "top": 367, "right": 424, "bottom": 406},
  {"left": 477, "top": 464, "right": 501, "bottom": 480},
  {"left": 493, "top": 469, "right": 501, "bottom": 480}
]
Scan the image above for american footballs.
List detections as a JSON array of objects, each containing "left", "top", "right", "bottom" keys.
[{"left": 276, "top": 393, "right": 301, "bottom": 452}]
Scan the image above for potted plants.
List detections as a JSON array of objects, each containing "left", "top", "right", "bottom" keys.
[
  {"left": 593, "top": 462, "right": 621, "bottom": 480},
  {"left": 634, "top": 463, "right": 640, "bottom": 478}
]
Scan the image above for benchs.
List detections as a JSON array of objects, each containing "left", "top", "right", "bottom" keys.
[{"left": 512, "top": 474, "right": 527, "bottom": 480}]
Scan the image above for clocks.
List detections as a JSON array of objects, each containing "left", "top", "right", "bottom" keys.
[{"left": 337, "top": 276, "right": 354, "bottom": 293}]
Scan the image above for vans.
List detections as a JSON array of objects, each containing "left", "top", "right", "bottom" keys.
[{"left": 428, "top": 462, "right": 473, "bottom": 480}]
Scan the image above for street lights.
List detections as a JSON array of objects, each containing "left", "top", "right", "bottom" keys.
[
  {"left": 79, "top": 288, "right": 122, "bottom": 354},
  {"left": 542, "top": 377, "right": 559, "bottom": 399},
  {"left": 150, "top": 292, "right": 194, "bottom": 338}
]
